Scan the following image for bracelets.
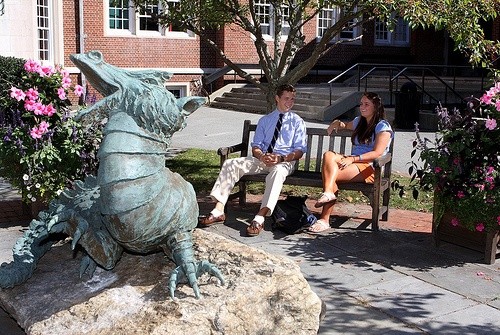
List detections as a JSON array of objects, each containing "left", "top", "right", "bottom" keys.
[
  {"left": 358, "top": 154, "right": 362, "bottom": 162},
  {"left": 344, "top": 123, "right": 347, "bottom": 128},
  {"left": 339, "top": 120, "right": 340, "bottom": 126},
  {"left": 280, "top": 156, "right": 284, "bottom": 162},
  {"left": 259, "top": 154, "right": 263, "bottom": 160}
]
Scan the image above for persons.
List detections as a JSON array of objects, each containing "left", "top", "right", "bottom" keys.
[
  {"left": 198, "top": 83, "right": 307, "bottom": 236},
  {"left": 309, "top": 93, "right": 393, "bottom": 232}
]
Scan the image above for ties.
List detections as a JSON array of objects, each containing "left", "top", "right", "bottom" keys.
[{"left": 265, "top": 113, "right": 284, "bottom": 154}]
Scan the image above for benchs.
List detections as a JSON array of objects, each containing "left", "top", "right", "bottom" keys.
[{"left": 218, "top": 120, "right": 395, "bottom": 234}]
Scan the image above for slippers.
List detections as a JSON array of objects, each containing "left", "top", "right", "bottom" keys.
[
  {"left": 309, "top": 220, "right": 331, "bottom": 232},
  {"left": 314, "top": 193, "right": 337, "bottom": 208}
]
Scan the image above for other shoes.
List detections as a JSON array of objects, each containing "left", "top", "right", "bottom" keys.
[
  {"left": 198, "top": 212, "right": 226, "bottom": 226},
  {"left": 246, "top": 222, "right": 265, "bottom": 235}
]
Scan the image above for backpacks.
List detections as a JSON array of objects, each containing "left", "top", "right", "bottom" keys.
[{"left": 270, "top": 195, "right": 317, "bottom": 235}]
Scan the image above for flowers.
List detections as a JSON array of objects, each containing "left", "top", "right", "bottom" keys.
[{"left": 409, "top": 78, "right": 500, "bottom": 235}]
